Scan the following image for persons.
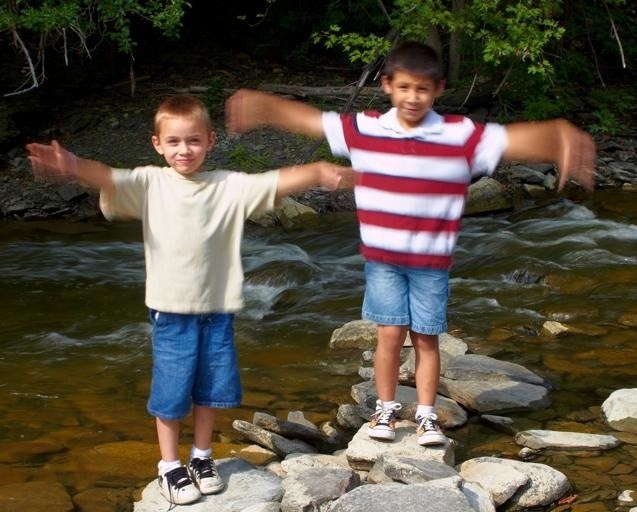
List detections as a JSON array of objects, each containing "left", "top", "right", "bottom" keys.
[
  {"left": 26, "top": 95, "right": 362, "bottom": 505},
  {"left": 226, "top": 38, "right": 598, "bottom": 447}
]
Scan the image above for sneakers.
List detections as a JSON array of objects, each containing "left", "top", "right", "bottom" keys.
[
  {"left": 188, "top": 456, "right": 224, "bottom": 495},
  {"left": 157, "top": 466, "right": 201, "bottom": 506},
  {"left": 415, "top": 414, "right": 446, "bottom": 445},
  {"left": 368, "top": 408, "right": 396, "bottom": 440}
]
